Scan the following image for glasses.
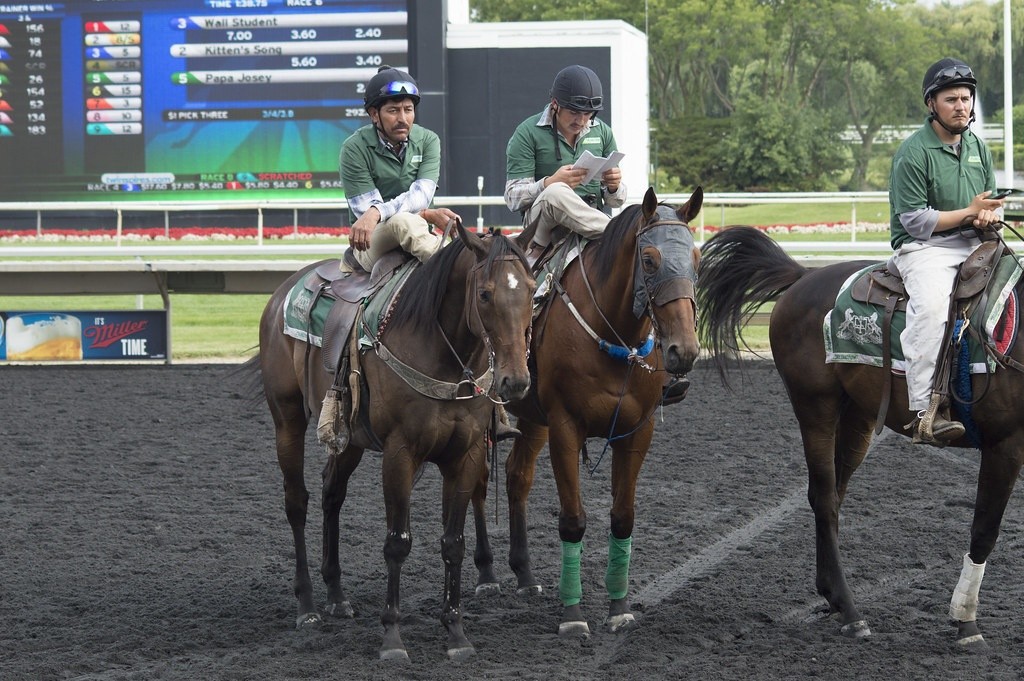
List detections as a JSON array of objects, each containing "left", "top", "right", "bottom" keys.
[
  {"left": 937, "top": 65, "right": 973, "bottom": 78},
  {"left": 379, "top": 80, "right": 419, "bottom": 95},
  {"left": 570, "top": 96, "right": 602, "bottom": 108}
]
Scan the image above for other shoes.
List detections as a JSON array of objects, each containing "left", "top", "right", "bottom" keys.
[
  {"left": 660, "top": 376, "right": 690, "bottom": 407},
  {"left": 490, "top": 397, "right": 522, "bottom": 442}
]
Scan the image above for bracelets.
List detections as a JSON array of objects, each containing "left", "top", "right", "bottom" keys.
[{"left": 424, "top": 209, "right": 426, "bottom": 217}]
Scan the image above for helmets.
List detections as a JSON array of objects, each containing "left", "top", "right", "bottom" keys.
[
  {"left": 548, "top": 64, "right": 604, "bottom": 111},
  {"left": 922, "top": 58, "right": 977, "bottom": 107},
  {"left": 364, "top": 69, "right": 421, "bottom": 110}
]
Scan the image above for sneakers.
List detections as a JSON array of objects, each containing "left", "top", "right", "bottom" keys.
[{"left": 912, "top": 411, "right": 966, "bottom": 444}]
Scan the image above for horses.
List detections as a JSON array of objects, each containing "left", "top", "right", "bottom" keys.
[
  {"left": 218, "top": 210, "right": 542, "bottom": 660},
  {"left": 470, "top": 186, "right": 703, "bottom": 636},
  {"left": 696, "top": 226, "right": 1023, "bottom": 652}
]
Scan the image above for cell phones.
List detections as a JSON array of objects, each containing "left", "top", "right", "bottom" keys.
[{"left": 993, "top": 190, "right": 1012, "bottom": 199}]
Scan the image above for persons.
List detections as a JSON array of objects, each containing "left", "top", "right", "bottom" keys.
[
  {"left": 890, "top": 58, "right": 1004, "bottom": 443},
  {"left": 503, "top": 64, "right": 628, "bottom": 248},
  {"left": 340, "top": 65, "right": 462, "bottom": 272}
]
